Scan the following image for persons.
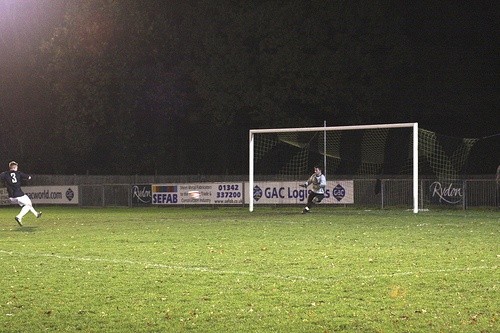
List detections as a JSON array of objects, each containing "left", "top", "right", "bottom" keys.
[
  {"left": 299, "top": 165, "right": 326, "bottom": 214},
  {"left": 0, "top": 160, "right": 42, "bottom": 226}
]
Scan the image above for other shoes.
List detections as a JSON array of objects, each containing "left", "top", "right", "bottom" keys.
[
  {"left": 301, "top": 209, "right": 306, "bottom": 213},
  {"left": 305, "top": 207, "right": 310, "bottom": 210}
]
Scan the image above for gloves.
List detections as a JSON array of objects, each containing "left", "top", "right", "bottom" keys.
[
  {"left": 299, "top": 184, "right": 306, "bottom": 187},
  {"left": 313, "top": 185, "right": 321, "bottom": 190}
]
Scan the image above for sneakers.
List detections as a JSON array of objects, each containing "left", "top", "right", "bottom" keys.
[
  {"left": 15, "top": 216, "right": 22, "bottom": 226},
  {"left": 36, "top": 211, "right": 42, "bottom": 218}
]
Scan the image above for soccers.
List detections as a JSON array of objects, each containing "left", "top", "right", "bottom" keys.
[{"left": 191, "top": 192, "right": 200, "bottom": 199}]
